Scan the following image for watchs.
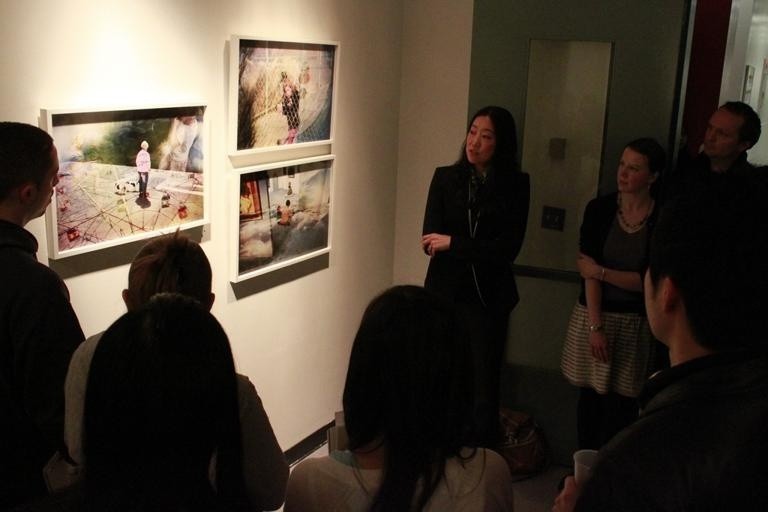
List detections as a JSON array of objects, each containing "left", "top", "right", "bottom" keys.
[{"left": 587, "top": 324, "right": 604, "bottom": 333}]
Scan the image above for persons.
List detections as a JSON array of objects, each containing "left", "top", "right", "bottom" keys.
[
  {"left": 0, "top": 122, "right": 86, "bottom": 512},
  {"left": 559, "top": 136, "right": 681, "bottom": 453},
  {"left": 5, "top": 291, "right": 247, "bottom": 511},
  {"left": 62, "top": 232, "right": 289, "bottom": 512},
  {"left": 283, "top": 284, "right": 514, "bottom": 511},
  {"left": 135, "top": 140, "right": 152, "bottom": 199},
  {"left": 277, "top": 184, "right": 293, "bottom": 225},
  {"left": 280, "top": 82, "right": 300, "bottom": 144},
  {"left": 668, "top": 101, "right": 761, "bottom": 184},
  {"left": 419, "top": 105, "right": 531, "bottom": 437},
  {"left": 550, "top": 184, "right": 766, "bottom": 511}
]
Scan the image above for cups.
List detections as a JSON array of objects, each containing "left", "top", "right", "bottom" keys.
[{"left": 572, "top": 448, "right": 596, "bottom": 486}]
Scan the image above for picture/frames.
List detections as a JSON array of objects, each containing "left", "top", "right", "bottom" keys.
[
  {"left": 40, "top": 102, "right": 211, "bottom": 260},
  {"left": 226, "top": 32, "right": 341, "bottom": 157},
  {"left": 231, "top": 154, "right": 334, "bottom": 286}
]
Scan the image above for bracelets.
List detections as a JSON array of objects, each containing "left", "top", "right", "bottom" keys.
[{"left": 601, "top": 262, "right": 607, "bottom": 281}]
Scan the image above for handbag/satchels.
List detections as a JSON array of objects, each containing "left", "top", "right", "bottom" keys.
[{"left": 493, "top": 406, "right": 549, "bottom": 484}]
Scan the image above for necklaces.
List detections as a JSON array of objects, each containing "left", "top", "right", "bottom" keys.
[{"left": 618, "top": 198, "right": 654, "bottom": 231}]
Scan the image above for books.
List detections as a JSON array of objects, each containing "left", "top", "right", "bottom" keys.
[{"left": 326, "top": 423, "right": 348, "bottom": 453}]
